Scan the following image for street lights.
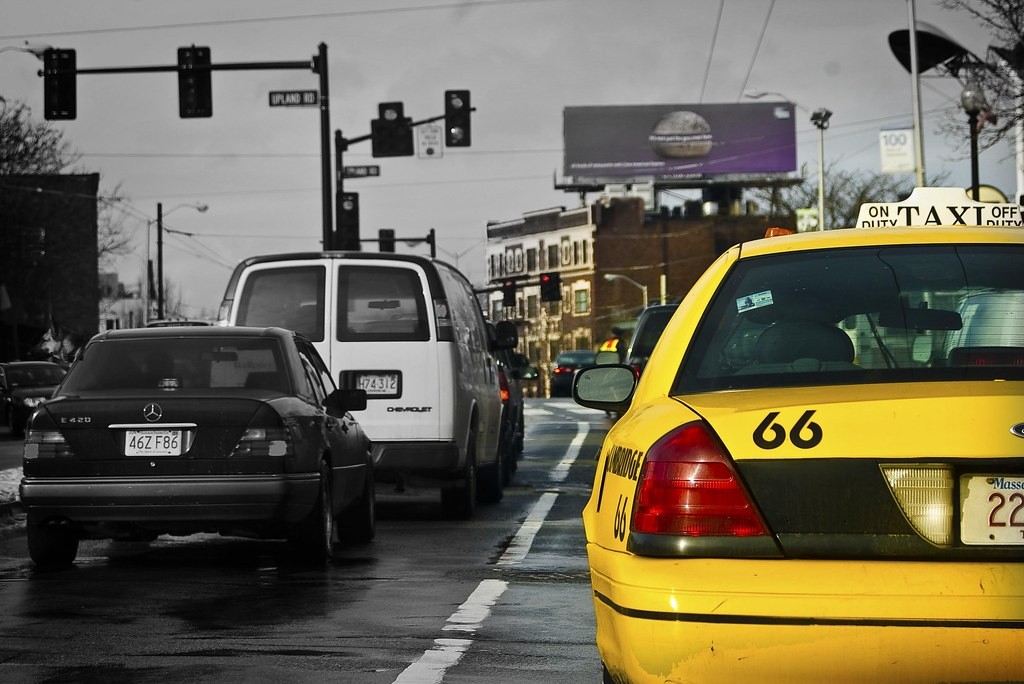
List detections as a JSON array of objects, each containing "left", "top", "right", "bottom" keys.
[
  {"left": 744, "top": 89, "right": 824, "bottom": 230},
  {"left": 961, "top": 82, "right": 985, "bottom": 202},
  {"left": 143, "top": 204, "right": 209, "bottom": 327},
  {"left": 604, "top": 274, "right": 648, "bottom": 309}
]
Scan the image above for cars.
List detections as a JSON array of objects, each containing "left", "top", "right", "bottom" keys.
[
  {"left": 0, "top": 361, "right": 66, "bottom": 440},
  {"left": 616, "top": 305, "right": 679, "bottom": 419},
  {"left": 486, "top": 320, "right": 542, "bottom": 471},
  {"left": 573, "top": 224, "right": 1024, "bottom": 683},
  {"left": 549, "top": 350, "right": 596, "bottom": 398},
  {"left": 20, "top": 325, "right": 377, "bottom": 568},
  {"left": 216, "top": 253, "right": 519, "bottom": 517}
]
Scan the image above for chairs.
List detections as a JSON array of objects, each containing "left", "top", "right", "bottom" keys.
[{"left": 754, "top": 319, "right": 855, "bottom": 365}]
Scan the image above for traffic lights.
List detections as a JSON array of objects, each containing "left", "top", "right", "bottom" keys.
[
  {"left": 378, "top": 101, "right": 404, "bottom": 157},
  {"left": 503, "top": 280, "right": 516, "bottom": 306},
  {"left": 539, "top": 272, "right": 552, "bottom": 302},
  {"left": 445, "top": 89, "right": 471, "bottom": 146},
  {"left": 336, "top": 192, "right": 360, "bottom": 251}
]
